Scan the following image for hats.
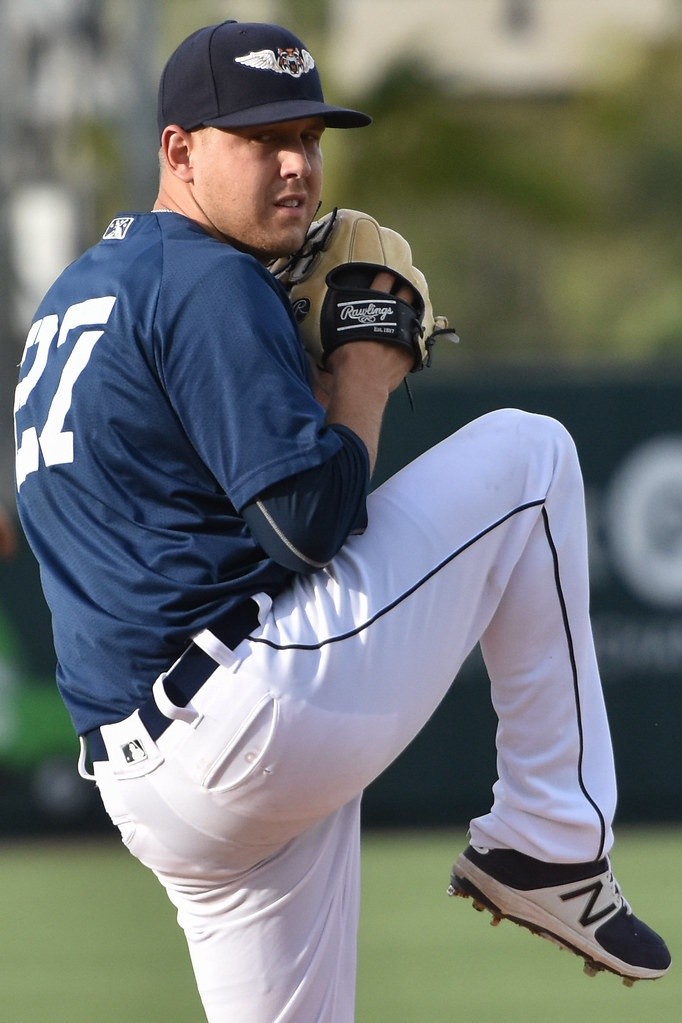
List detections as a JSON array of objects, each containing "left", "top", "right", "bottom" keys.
[{"left": 158, "top": 20, "right": 373, "bottom": 136}]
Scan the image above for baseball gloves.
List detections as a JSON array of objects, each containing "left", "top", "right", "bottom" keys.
[{"left": 264, "top": 206, "right": 452, "bottom": 367}]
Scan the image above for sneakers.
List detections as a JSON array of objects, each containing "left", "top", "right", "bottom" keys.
[{"left": 445, "top": 843, "right": 673, "bottom": 987}]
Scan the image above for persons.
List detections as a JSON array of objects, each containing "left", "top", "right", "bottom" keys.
[{"left": 12, "top": 20, "right": 673, "bottom": 1023}]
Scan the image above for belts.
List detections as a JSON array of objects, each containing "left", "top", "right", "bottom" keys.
[{"left": 83, "top": 598, "right": 261, "bottom": 761}]
